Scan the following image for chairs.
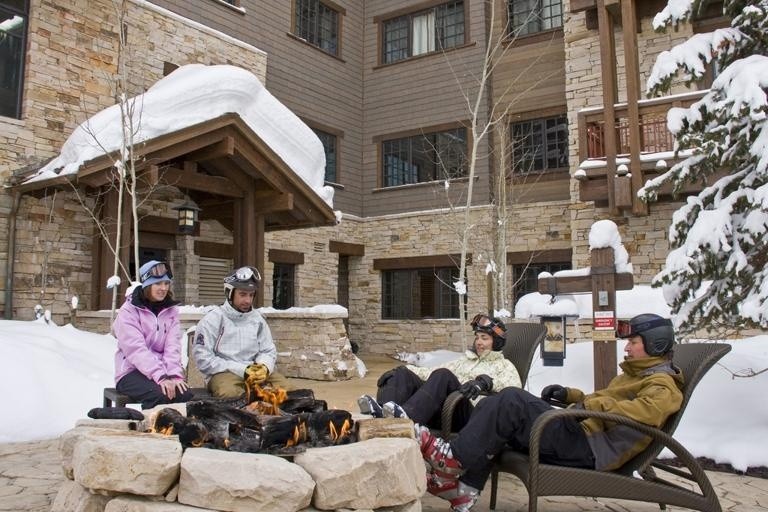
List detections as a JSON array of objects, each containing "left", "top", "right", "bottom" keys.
[
  {"left": 490, "top": 343, "right": 732, "bottom": 512},
  {"left": 376, "top": 323, "right": 547, "bottom": 442}
]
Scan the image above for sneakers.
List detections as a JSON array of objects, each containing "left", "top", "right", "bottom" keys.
[
  {"left": 420, "top": 428, "right": 463, "bottom": 479},
  {"left": 359, "top": 396, "right": 382, "bottom": 419},
  {"left": 383, "top": 403, "right": 408, "bottom": 420},
  {"left": 426, "top": 469, "right": 480, "bottom": 512}
]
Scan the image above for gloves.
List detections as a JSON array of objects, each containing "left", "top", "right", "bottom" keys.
[
  {"left": 568, "top": 401, "right": 590, "bottom": 421},
  {"left": 377, "top": 366, "right": 404, "bottom": 388},
  {"left": 541, "top": 385, "right": 567, "bottom": 403},
  {"left": 255, "top": 364, "right": 267, "bottom": 384},
  {"left": 245, "top": 365, "right": 259, "bottom": 384},
  {"left": 459, "top": 375, "right": 493, "bottom": 399}
]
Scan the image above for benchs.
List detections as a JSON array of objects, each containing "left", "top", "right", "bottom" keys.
[{"left": 103, "top": 387, "right": 219, "bottom": 408}]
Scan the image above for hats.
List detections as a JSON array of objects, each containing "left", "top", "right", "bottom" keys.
[{"left": 139, "top": 261, "right": 172, "bottom": 288}]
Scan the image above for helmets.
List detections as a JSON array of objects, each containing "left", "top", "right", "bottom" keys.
[
  {"left": 473, "top": 316, "right": 507, "bottom": 350},
  {"left": 624, "top": 314, "right": 674, "bottom": 355},
  {"left": 223, "top": 267, "right": 260, "bottom": 301}
]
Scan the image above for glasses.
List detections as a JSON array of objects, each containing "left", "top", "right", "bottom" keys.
[
  {"left": 224, "top": 266, "right": 261, "bottom": 283},
  {"left": 617, "top": 318, "right": 672, "bottom": 339},
  {"left": 470, "top": 315, "right": 505, "bottom": 337},
  {"left": 140, "top": 262, "right": 172, "bottom": 282}
]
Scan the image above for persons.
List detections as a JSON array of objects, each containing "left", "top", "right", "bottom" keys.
[
  {"left": 358, "top": 315, "right": 522, "bottom": 434},
  {"left": 113, "top": 260, "right": 191, "bottom": 409},
  {"left": 416, "top": 314, "right": 684, "bottom": 511},
  {"left": 191, "top": 267, "right": 293, "bottom": 400}
]
